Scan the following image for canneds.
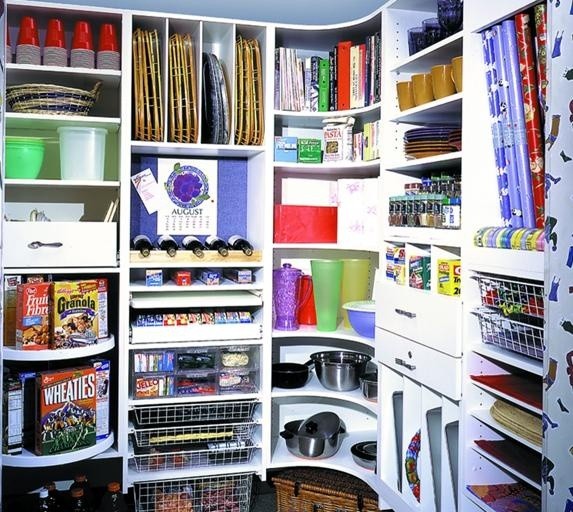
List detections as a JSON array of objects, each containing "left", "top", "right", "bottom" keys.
[{"left": 389, "top": 172, "right": 461, "bottom": 230}]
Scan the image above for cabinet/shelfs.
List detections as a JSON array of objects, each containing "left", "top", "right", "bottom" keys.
[{"left": 2, "top": 0, "right": 571, "bottom": 510}]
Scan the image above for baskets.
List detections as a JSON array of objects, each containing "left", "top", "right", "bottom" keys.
[{"left": 7, "top": 81, "right": 100, "bottom": 117}]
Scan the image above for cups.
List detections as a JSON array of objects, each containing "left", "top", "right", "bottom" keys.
[
  {"left": 431, "top": 64, "right": 455, "bottom": 100},
  {"left": 7, "top": 16, "right": 120, "bottom": 69},
  {"left": 451, "top": 56, "right": 462, "bottom": 93},
  {"left": 397, "top": 81, "right": 415, "bottom": 112},
  {"left": 422, "top": 18, "right": 448, "bottom": 48},
  {"left": 310, "top": 259, "right": 343, "bottom": 332},
  {"left": 408, "top": 26, "right": 426, "bottom": 56},
  {"left": 341, "top": 258, "right": 369, "bottom": 328},
  {"left": 412, "top": 74, "right": 434, "bottom": 106},
  {"left": 273, "top": 263, "right": 317, "bottom": 330},
  {"left": 437, "top": 0, "right": 463, "bottom": 34}
]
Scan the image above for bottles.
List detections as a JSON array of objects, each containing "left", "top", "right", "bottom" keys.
[
  {"left": 388, "top": 172, "right": 461, "bottom": 228},
  {"left": 181, "top": 235, "right": 204, "bottom": 257},
  {"left": 205, "top": 235, "right": 228, "bottom": 257},
  {"left": 227, "top": 234, "right": 254, "bottom": 256},
  {"left": 38, "top": 473, "right": 93, "bottom": 512},
  {"left": 133, "top": 235, "right": 153, "bottom": 257},
  {"left": 157, "top": 235, "right": 179, "bottom": 257},
  {"left": 101, "top": 482, "right": 129, "bottom": 512}
]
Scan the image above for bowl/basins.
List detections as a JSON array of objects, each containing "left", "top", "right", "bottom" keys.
[
  {"left": 5, "top": 135, "right": 46, "bottom": 178},
  {"left": 309, "top": 351, "right": 372, "bottom": 392},
  {"left": 342, "top": 299, "right": 375, "bottom": 339}
]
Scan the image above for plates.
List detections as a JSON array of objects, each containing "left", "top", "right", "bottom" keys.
[{"left": 403, "top": 126, "right": 461, "bottom": 159}]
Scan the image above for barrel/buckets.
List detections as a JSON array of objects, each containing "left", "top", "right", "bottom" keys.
[
  {"left": 6, "top": 136, "right": 45, "bottom": 178},
  {"left": 56, "top": 126, "right": 108, "bottom": 182}
]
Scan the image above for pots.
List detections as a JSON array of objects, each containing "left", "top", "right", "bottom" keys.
[
  {"left": 272, "top": 359, "right": 313, "bottom": 389},
  {"left": 350, "top": 440, "right": 377, "bottom": 474},
  {"left": 359, "top": 369, "right": 378, "bottom": 402},
  {"left": 279, "top": 411, "right": 346, "bottom": 460}
]
